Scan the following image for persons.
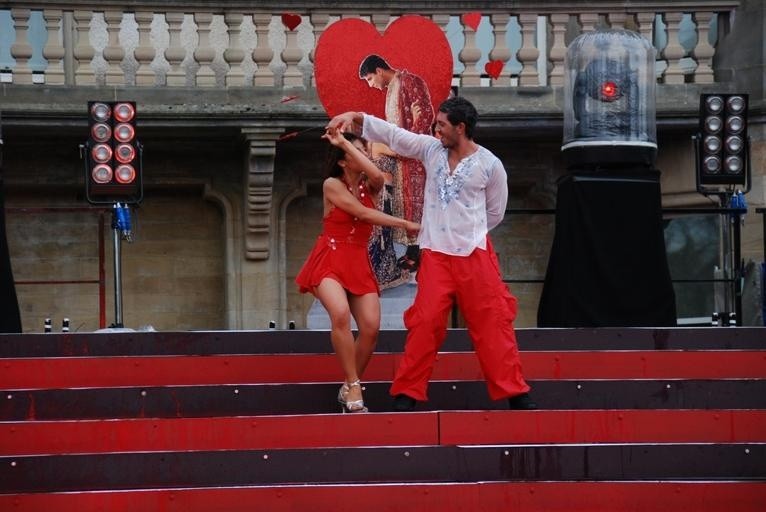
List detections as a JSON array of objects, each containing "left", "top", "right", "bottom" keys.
[
  {"left": 357, "top": 54, "right": 437, "bottom": 272},
  {"left": 366, "top": 137, "right": 408, "bottom": 292},
  {"left": 294, "top": 129, "right": 424, "bottom": 414},
  {"left": 325, "top": 95, "right": 540, "bottom": 413}
]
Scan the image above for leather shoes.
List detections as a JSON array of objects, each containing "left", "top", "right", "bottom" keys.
[
  {"left": 392, "top": 394, "right": 417, "bottom": 411},
  {"left": 508, "top": 394, "right": 536, "bottom": 409}
]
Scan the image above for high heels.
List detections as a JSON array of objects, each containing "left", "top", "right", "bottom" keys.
[
  {"left": 337, "top": 386, "right": 369, "bottom": 413},
  {"left": 345, "top": 379, "right": 363, "bottom": 411}
]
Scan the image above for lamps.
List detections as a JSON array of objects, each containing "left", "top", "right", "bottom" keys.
[
  {"left": 42, "top": 316, "right": 71, "bottom": 332},
  {"left": 710, "top": 311, "right": 739, "bottom": 325},
  {"left": 573, "top": 57, "right": 641, "bottom": 141},
  {"left": 688, "top": 92, "right": 754, "bottom": 325},
  {"left": 80, "top": 100, "right": 145, "bottom": 333},
  {"left": 268, "top": 319, "right": 298, "bottom": 329}
]
